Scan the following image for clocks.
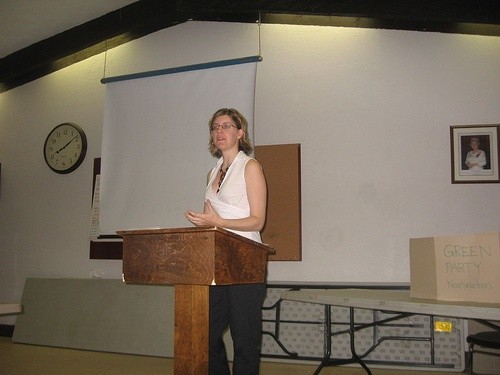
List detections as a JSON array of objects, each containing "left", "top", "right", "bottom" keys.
[{"left": 44, "top": 123, "right": 87, "bottom": 174}]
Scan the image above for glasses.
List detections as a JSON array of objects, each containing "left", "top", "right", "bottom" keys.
[{"left": 212, "top": 124, "right": 238, "bottom": 129}]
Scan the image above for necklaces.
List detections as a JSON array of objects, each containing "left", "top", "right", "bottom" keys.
[{"left": 217, "top": 163, "right": 230, "bottom": 192}]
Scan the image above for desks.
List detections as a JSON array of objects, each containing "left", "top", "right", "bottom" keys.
[{"left": 281, "top": 289, "right": 500, "bottom": 375}]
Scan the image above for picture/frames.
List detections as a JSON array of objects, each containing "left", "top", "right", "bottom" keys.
[{"left": 450, "top": 124, "right": 500, "bottom": 185}]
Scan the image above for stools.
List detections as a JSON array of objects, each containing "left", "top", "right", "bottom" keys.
[{"left": 466, "top": 331, "right": 500, "bottom": 375}]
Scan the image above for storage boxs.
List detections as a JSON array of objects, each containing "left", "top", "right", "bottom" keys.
[{"left": 409, "top": 234, "right": 500, "bottom": 303}]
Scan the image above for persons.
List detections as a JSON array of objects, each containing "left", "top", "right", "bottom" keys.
[
  {"left": 465, "top": 137, "right": 486, "bottom": 170},
  {"left": 185, "top": 108, "right": 267, "bottom": 375}
]
